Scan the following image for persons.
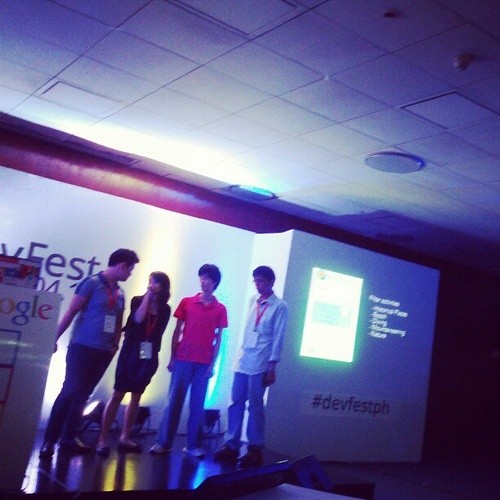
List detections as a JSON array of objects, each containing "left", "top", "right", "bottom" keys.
[
  {"left": 97, "top": 272, "right": 171, "bottom": 456},
  {"left": 150, "top": 264, "right": 229, "bottom": 458},
  {"left": 36, "top": 249, "right": 139, "bottom": 455},
  {"left": 220, "top": 266, "right": 288, "bottom": 465}
]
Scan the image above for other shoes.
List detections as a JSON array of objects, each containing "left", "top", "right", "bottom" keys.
[
  {"left": 213, "top": 445, "right": 240, "bottom": 460},
  {"left": 59, "top": 438, "right": 94, "bottom": 453},
  {"left": 181, "top": 446, "right": 206, "bottom": 458},
  {"left": 148, "top": 443, "right": 175, "bottom": 454},
  {"left": 118, "top": 439, "right": 143, "bottom": 453},
  {"left": 95, "top": 443, "right": 110, "bottom": 457},
  {"left": 39, "top": 440, "right": 56, "bottom": 458},
  {"left": 236, "top": 446, "right": 265, "bottom": 467}
]
